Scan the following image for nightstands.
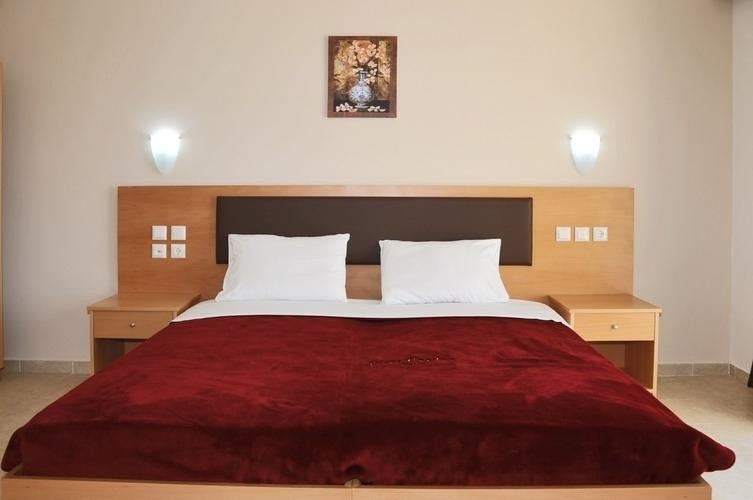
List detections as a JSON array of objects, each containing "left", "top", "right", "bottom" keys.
[
  {"left": 85, "top": 291, "right": 201, "bottom": 375},
  {"left": 547, "top": 293, "right": 661, "bottom": 399}
]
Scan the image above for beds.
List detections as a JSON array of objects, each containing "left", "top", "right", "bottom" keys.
[{"left": 0, "top": 297, "right": 713, "bottom": 500}]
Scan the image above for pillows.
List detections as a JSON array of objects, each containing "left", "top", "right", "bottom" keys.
[{"left": 214, "top": 233, "right": 510, "bottom": 303}]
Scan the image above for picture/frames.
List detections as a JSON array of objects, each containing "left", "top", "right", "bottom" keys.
[{"left": 327, "top": 35, "right": 397, "bottom": 118}]
